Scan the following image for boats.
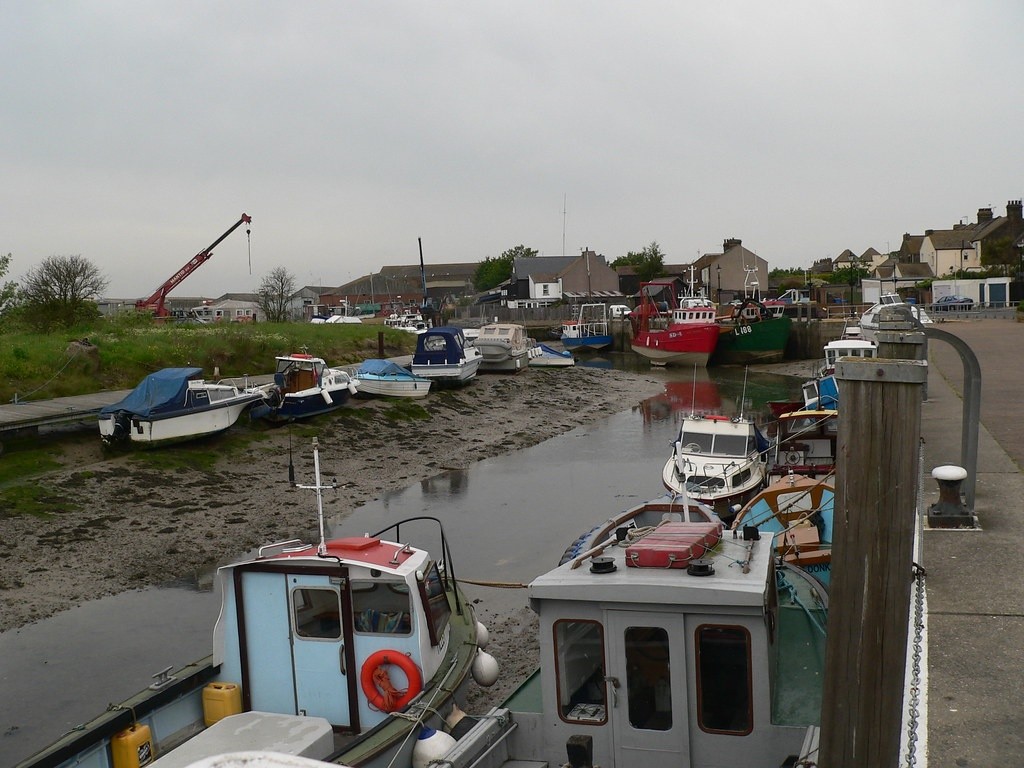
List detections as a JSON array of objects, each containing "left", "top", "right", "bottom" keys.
[
  {"left": 244, "top": 345, "right": 363, "bottom": 427},
  {"left": 469, "top": 323, "right": 534, "bottom": 374},
  {"left": 490, "top": 292, "right": 932, "bottom": 767},
  {"left": 714, "top": 247, "right": 794, "bottom": 367},
  {"left": 384, "top": 308, "right": 430, "bottom": 335},
  {"left": 638, "top": 265, "right": 718, "bottom": 371},
  {"left": 412, "top": 326, "right": 487, "bottom": 386},
  {"left": 350, "top": 357, "right": 435, "bottom": 401},
  {"left": 11, "top": 435, "right": 497, "bottom": 766},
  {"left": 525, "top": 342, "right": 583, "bottom": 371},
  {"left": 96, "top": 364, "right": 268, "bottom": 454}
]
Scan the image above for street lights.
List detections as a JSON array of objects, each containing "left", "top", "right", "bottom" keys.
[
  {"left": 1017, "top": 240, "right": 1024, "bottom": 301},
  {"left": 848, "top": 251, "right": 860, "bottom": 320},
  {"left": 715, "top": 263, "right": 723, "bottom": 306}
]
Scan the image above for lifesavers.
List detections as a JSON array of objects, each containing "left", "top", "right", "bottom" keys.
[
  {"left": 291, "top": 354, "right": 312, "bottom": 358},
  {"left": 361, "top": 649, "right": 421, "bottom": 715},
  {"left": 383, "top": 309, "right": 387, "bottom": 315},
  {"left": 706, "top": 416, "right": 729, "bottom": 421}
]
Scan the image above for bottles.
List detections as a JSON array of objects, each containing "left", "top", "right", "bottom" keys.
[
  {"left": 111, "top": 722, "right": 154, "bottom": 768},
  {"left": 202, "top": 682, "right": 242, "bottom": 727}
]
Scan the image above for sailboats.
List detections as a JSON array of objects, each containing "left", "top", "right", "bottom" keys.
[
  {"left": 556, "top": 247, "right": 615, "bottom": 350},
  {"left": 307, "top": 272, "right": 380, "bottom": 325}
]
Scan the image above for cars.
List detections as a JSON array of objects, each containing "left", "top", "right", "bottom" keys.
[
  {"left": 928, "top": 295, "right": 973, "bottom": 312},
  {"left": 627, "top": 302, "right": 652, "bottom": 318}
]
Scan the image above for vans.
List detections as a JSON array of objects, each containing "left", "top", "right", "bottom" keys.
[{"left": 608, "top": 303, "right": 632, "bottom": 320}]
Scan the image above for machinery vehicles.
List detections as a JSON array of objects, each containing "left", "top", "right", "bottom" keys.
[{"left": 132, "top": 211, "right": 256, "bottom": 326}]
